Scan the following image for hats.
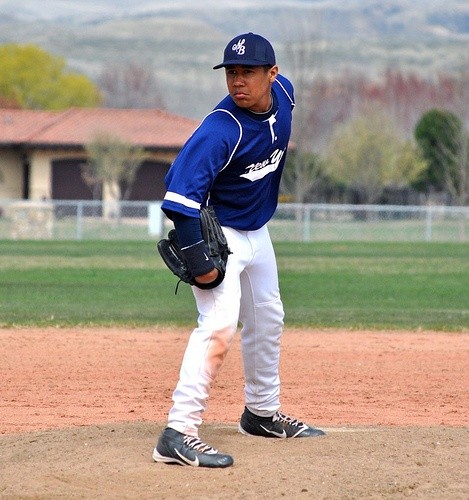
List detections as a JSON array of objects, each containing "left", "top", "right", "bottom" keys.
[{"left": 213, "top": 31, "right": 276, "bottom": 70}]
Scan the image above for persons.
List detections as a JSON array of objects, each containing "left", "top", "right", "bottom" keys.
[{"left": 151, "top": 32, "right": 333, "bottom": 468}]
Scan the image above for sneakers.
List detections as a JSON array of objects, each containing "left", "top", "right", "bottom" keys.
[
  {"left": 239, "top": 407, "right": 326, "bottom": 439},
  {"left": 152, "top": 428, "right": 233, "bottom": 467}
]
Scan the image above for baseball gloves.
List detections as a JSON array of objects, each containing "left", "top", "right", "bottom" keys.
[{"left": 155, "top": 206, "right": 231, "bottom": 290}]
